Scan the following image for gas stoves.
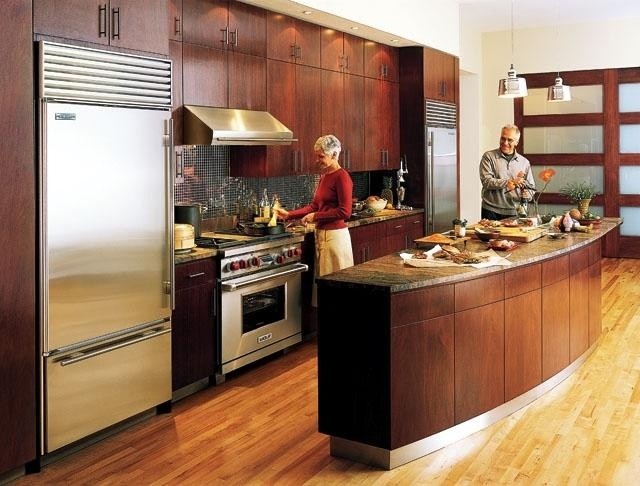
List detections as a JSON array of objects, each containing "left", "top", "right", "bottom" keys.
[{"left": 194, "top": 226, "right": 294, "bottom": 249}]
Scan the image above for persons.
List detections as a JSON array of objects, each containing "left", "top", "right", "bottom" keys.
[
  {"left": 479, "top": 124, "right": 536, "bottom": 221},
  {"left": 274, "top": 134, "right": 355, "bottom": 310}
]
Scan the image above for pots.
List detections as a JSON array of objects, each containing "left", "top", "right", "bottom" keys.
[{"left": 243, "top": 218, "right": 295, "bottom": 236}]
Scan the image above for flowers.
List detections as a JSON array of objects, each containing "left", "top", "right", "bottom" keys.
[{"left": 503, "top": 166, "right": 556, "bottom": 225}]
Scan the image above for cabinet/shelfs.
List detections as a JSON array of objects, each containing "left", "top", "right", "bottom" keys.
[
  {"left": 167, "top": 258, "right": 217, "bottom": 394},
  {"left": 168, "top": 2, "right": 402, "bottom": 177},
  {"left": 33, "top": 0, "right": 170, "bottom": 57},
  {"left": 422, "top": 48, "right": 456, "bottom": 104},
  {"left": 347, "top": 211, "right": 424, "bottom": 267}
]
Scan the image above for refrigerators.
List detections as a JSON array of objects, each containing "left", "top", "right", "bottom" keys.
[{"left": 37, "top": 39, "right": 177, "bottom": 460}]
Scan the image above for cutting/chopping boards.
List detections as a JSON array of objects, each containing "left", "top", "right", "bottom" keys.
[
  {"left": 465, "top": 223, "right": 550, "bottom": 243},
  {"left": 473, "top": 229, "right": 500, "bottom": 243}
]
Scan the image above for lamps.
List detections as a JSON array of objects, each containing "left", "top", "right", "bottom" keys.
[{"left": 498, "top": 0, "right": 572, "bottom": 103}]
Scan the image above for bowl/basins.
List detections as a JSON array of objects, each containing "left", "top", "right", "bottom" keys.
[{"left": 364, "top": 199, "right": 388, "bottom": 213}]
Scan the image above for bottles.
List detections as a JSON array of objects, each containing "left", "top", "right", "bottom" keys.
[
  {"left": 258, "top": 189, "right": 281, "bottom": 220},
  {"left": 519, "top": 187, "right": 530, "bottom": 219}
]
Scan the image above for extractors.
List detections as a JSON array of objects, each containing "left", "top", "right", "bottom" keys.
[{"left": 182, "top": 105, "right": 300, "bottom": 148}]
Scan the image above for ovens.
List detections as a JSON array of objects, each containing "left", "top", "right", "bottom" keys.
[{"left": 218, "top": 242, "right": 311, "bottom": 374}]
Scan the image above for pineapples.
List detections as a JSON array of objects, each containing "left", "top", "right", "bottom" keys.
[{"left": 380, "top": 176, "right": 393, "bottom": 205}]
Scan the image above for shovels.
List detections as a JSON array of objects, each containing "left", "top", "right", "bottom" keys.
[{"left": 267, "top": 201, "right": 279, "bottom": 227}]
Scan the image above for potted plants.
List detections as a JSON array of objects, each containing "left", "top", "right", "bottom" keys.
[
  {"left": 452, "top": 219, "right": 468, "bottom": 237},
  {"left": 558, "top": 181, "right": 601, "bottom": 216}
]
